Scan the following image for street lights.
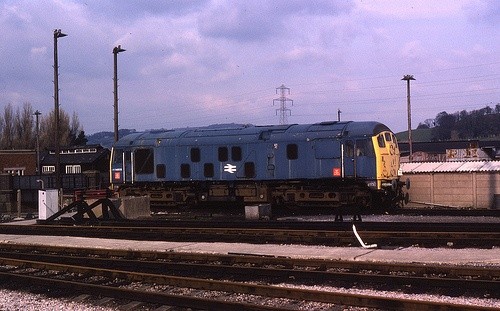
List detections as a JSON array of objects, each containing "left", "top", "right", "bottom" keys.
[
  {"left": 52, "top": 29, "right": 68, "bottom": 189},
  {"left": 32, "top": 110, "right": 42, "bottom": 173},
  {"left": 401, "top": 74, "right": 417, "bottom": 163},
  {"left": 113, "top": 46, "right": 126, "bottom": 143}
]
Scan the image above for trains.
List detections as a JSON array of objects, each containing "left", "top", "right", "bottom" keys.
[{"left": 108, "top": 108, "right": 411, "bottom": 222}]
